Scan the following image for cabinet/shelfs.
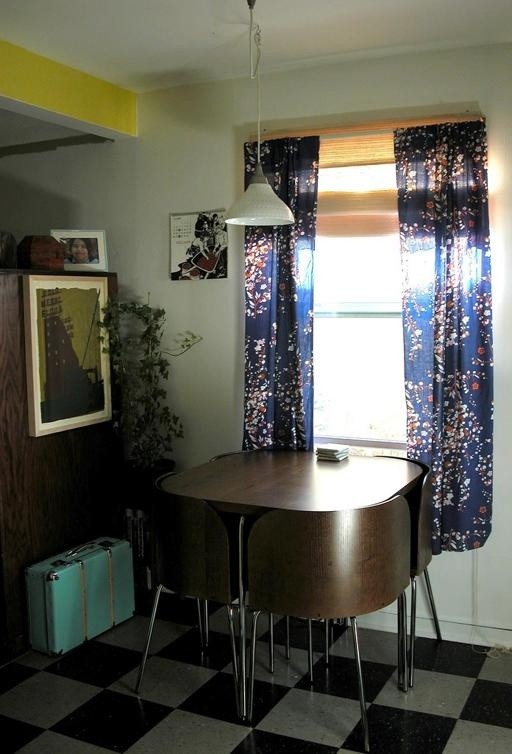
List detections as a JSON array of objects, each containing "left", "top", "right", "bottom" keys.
[{"left": 0, "top": 268, "right": 118, "bottom": 635}]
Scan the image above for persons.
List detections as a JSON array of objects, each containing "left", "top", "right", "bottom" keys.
[{"left": 63, "top": 237, "right": 98, "bottom": 263}]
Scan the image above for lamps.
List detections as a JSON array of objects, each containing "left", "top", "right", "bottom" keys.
[{"left": 225, "top": 0, "right": 296, "bottom": 227}]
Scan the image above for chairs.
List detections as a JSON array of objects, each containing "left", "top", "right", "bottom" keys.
[
  {"left": 134, "top": 472, "right": 242, "bottom": 721},
  {"left": 375, "top": 453, "right": 443, "bottom": 687},
  {"left": 246, "top": 496, "right": 412, "bottom": 751}
]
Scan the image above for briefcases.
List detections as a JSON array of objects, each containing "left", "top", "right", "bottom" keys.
[{"left": 25, "top": 536, "right": 138, "bottom": 662}]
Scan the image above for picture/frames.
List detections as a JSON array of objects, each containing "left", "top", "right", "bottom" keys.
[{"left": 23, "top": 228, "right": 113, "bottom": 439}]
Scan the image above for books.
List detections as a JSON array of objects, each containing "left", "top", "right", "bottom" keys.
[{"left": 315, "top": 443, "right": 349, "bottom": 461}]
